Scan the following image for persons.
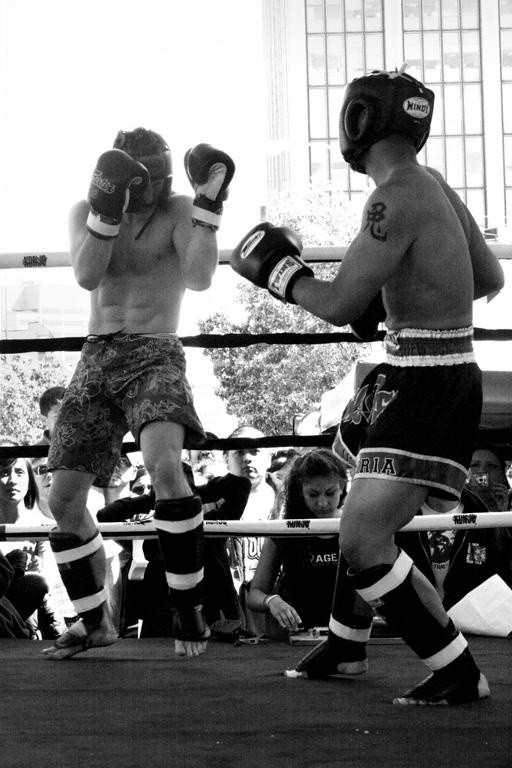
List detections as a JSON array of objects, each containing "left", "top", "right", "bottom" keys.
[
  {"left": 1, "top": 385, "right": 510, "bottom": 648},
  {"left": 42, "top": 123, "right": 237, "bottom": 662},
  {"left": 227, "top": 66, "right": 508, "bottom": 711}
]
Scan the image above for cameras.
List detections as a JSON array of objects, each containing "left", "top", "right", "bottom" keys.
[{"left": 467, "top": 473, "right": 489, "bottom": 488}]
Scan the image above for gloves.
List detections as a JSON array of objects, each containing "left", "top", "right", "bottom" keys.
[
  {"left": 84, "top": 149, "right": 149, "bottom": 241},
  {"left": 230, "top": 222, "right": 314, "bottom": 306},
  {"left": 184, "top": 144, "right": 235, "bottom": 231},
  {"left": 350, "top": 290, "right": 386, "bottom": 341}
]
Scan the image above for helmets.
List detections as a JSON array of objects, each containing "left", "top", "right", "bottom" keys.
[
  {"left": 339, "top": 64, "right": 434, "bottom": 174},
  {"left": 113, "top": 128, "right": 173, "bottom": 213}
]
